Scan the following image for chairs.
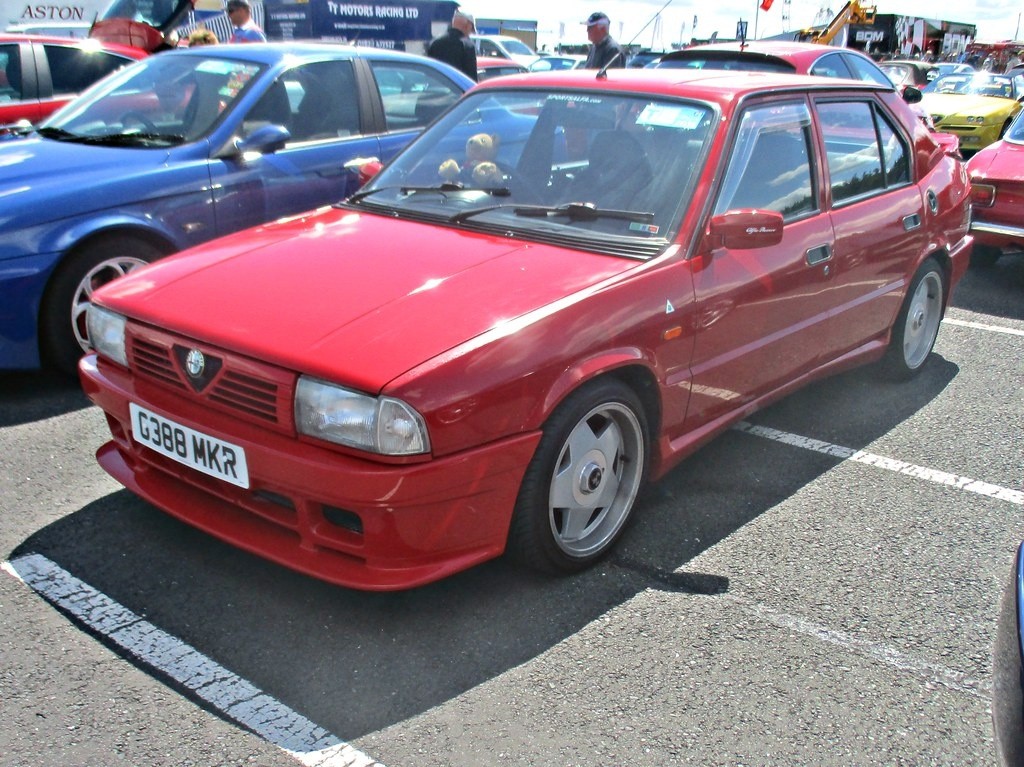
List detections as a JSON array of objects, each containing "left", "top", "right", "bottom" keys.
[
  {"left": 5, "top": 56, "right": 22, "bottom": 93},
  {"left": 727, "top": 129, "right": 803, "bottom": 215},
  {"left": 562, "top": 130, "right": 652, "bottom": 214},
  {"left": 296, "top": 74, "right": 354, "bottom": 136},
  {"left": 237, "top": 78, "right": 290, "bottom": 129}
]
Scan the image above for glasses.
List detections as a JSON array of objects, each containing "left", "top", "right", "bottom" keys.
[{"left": 227, "top": 9, "right": 236, "bottom": 14}]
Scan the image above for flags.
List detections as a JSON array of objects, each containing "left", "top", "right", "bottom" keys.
[{"left": 761, "top": 0, "right": 773, "bottom": 10}]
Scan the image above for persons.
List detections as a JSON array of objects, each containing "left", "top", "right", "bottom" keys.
[
  {"left": 580, "top": 12, "right": 626, "bottom": 69},
  {"left": 428, "top": 14, "right": 478, "bottom": 84},
  {"left": 151, "top": 0, "right": 268, "bottom": 108},
  {"left": 873, "top": 47, "right": 1024, "bottom": 75}
]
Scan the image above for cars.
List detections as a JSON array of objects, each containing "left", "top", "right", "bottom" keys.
[
  {"left": 966, "top": 102, "right": 1024, "bottom": 270},
  {"left": 457, "top": 31, "right": 667, "bottom": 120},
  {"left": 0, "top": 31, "right": 235, "bottom": 129},
  {"left": 878, "top": 59, "right": 1024, "bottom": 155},
  {"left": 0, "top": 42, "right": 572, "bottom": 380},
  {"left": 614, "top": 39, "right": 933, "bottom": 143},
  {"left": 78, "top": 67, "right": 978, "bottom": 592}
]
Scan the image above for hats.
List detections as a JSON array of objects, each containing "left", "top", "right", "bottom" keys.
[
  {"left": 580, "top": 12, "right": 610, "bottom": 26},
  {"left": 455, "top": 9, "right": 478, "bottom": 34}
]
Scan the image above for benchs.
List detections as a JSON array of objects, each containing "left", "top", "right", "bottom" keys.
[{"left": 382, "top": 91, "right": 454, "bottom": 131}]
